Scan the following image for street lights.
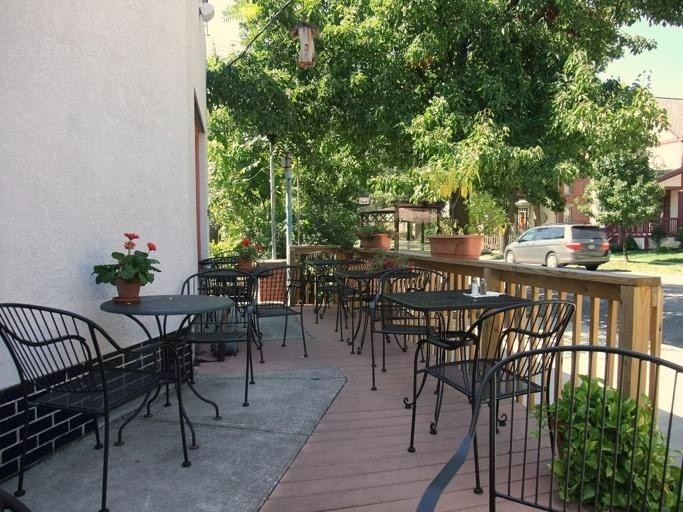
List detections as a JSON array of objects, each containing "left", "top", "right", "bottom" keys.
[{"left": 284, "top": 150, "right": 295, "bottom": 306}]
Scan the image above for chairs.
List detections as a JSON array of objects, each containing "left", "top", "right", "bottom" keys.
[
  {"left": 337, "top": 259, "right": 388, "bottom": 333},
  {"left": 410, "top": 301, "right": 578, "bottom": 495},
  {"left": 370, "top": 266, "right": 451, "bottom": 391},
  {"left": 0, "top": 303, "right": 189, "bottom": 512},
  {"left": 415, "top": 346, "right": 683, "bottom": 512},
  {"left": 347, "top": 266, "right": 424, "bottom": 354},
  {"left": 166, "top": 269, "right": 256, "bottom": 408},
  {"left": 251, "top": 266, "right": 309, "bottom": 357}
]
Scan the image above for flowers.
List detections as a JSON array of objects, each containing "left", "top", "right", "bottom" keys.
[
  {"left": 233, "top": 238, "right": 264, "bottom": 260},
  {"left": 89, "top": 232, "right": 161, "bottom": 287}
]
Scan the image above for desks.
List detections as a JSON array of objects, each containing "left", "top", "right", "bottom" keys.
[
  {"left": 380, "top": 290, "right": 532, "bottom": 402},
  {"left": 99, "top": 294, "right": 235, "bottom": 468},
  {"left": 308, "top": 258, "right": 366, "bottom": 311},
  {"left": 200, "top": 264, "right": 287, "bottom": 347},
  {"left": 340, "top": 269, "right": 419, "bottom": 345}
]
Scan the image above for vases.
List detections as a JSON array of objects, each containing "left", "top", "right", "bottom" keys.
[
  {"left": 113, "top": 277, "right": 141, "bottom": 299},
  {"left": 236, "top": 258, "right": 252, "bottom": 271}
]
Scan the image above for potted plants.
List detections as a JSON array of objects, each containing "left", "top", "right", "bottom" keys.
[
  {"left": 357, "top": 226, "right": 394, "bottom": 249},
  {"left": 409, "top": 155, "right": 511, "bottom": 258}
]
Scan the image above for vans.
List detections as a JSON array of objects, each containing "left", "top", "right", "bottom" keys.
[{"left": 504, "top": 224, "right": 610, "bottom": 270}]
